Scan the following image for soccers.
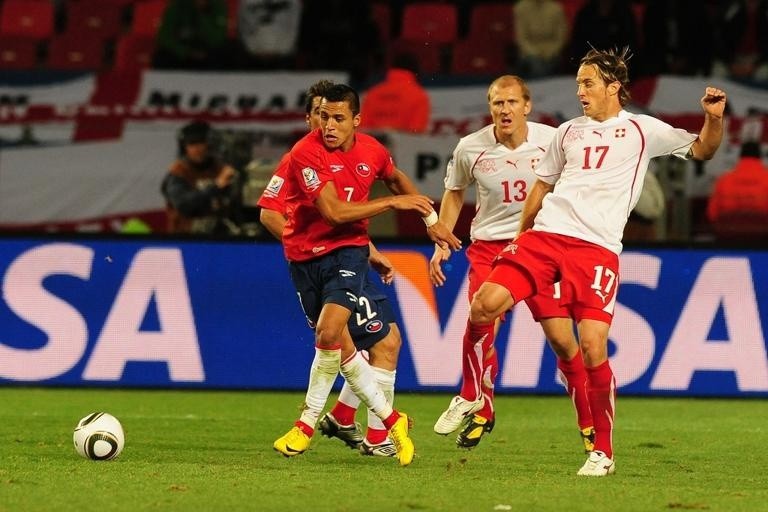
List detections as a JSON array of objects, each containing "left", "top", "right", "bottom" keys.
[{"left": 73, "top": 411, "right": 125, "bottom": 460}]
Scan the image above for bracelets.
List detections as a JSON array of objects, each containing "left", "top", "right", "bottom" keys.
[{"left": 422, "top": 209, "right": 437, "bottom": 228}]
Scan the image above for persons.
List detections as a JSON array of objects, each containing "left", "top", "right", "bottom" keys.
[
  {"left": 271, "top": 85, "right": 466, "bottom": 468},
  {"left": 162, "top": 121, "right": 244, "bottom": 240},
  {"left": 432, "top": 43, "right": 728, "bottom": 478},
  {"left": 364, "top": 130, "right": 400, "bottom": 237},
  {"left": 155, "top": 2, "right": 380, "bottom": 76},
  {"left": 425, "top": 69, "right": 604, "bottom": 455},
  {"left": 510, "top": 2, "right": 767, "bottom": 89},
  {"left": 626, "top": 142, "right": 767, "bottom": 245},
  {"left": 252, "top": 78, "right": 413, "bottom": 459}
]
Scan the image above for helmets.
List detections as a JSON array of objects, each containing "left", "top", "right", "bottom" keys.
[{"left": 175, "top": 119, "right": 214, "bottom": 161}]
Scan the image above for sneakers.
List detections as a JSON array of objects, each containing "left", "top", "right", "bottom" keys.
[
  {"left": 455, "top": 413, "right": 495, "bottom": 447},
  {"left": 359, "top": 438, "right": 416, "bottom": 458},
  {"left": 383, "top": 409, "right": 415, "bottom": 467},
  {"left": 433, "top": 395, "right": 485, "bottom": 437},
  {"left": 576, "top": 448, "right": 615, "bottom": 477},
  {"left": 274, "top": 420, "right": 315, "bottom": 459},
  {"left": 580, "top": 425, "right": 596, "bottom": 451},
  {"left": 319, "top": 412, "right": 367, "bottom": 451}
]
